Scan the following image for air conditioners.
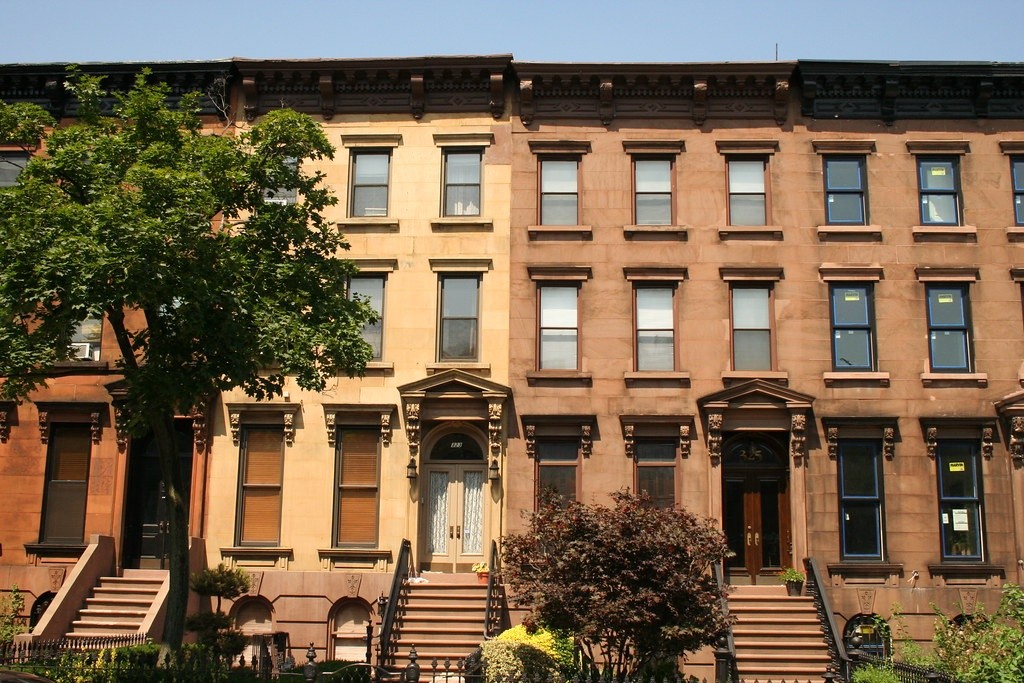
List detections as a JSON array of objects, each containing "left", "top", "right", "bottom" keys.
[{"left": 71, "top": 342, "right": 94, "bottom": 361}]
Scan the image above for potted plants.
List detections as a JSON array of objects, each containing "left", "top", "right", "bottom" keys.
[
  {"left": 778, "top": 567, "right": 804, "bottom": 596},
  {"left": 471, "top": 561, "right": 491, "bottom": 584}
]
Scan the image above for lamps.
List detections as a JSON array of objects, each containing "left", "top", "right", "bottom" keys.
[
  {"left": 489, "top": 460, "right": 499, "bottom": 479},
  {"left": 407, "top": 458, "right": 417, "bottom": 478}
]
